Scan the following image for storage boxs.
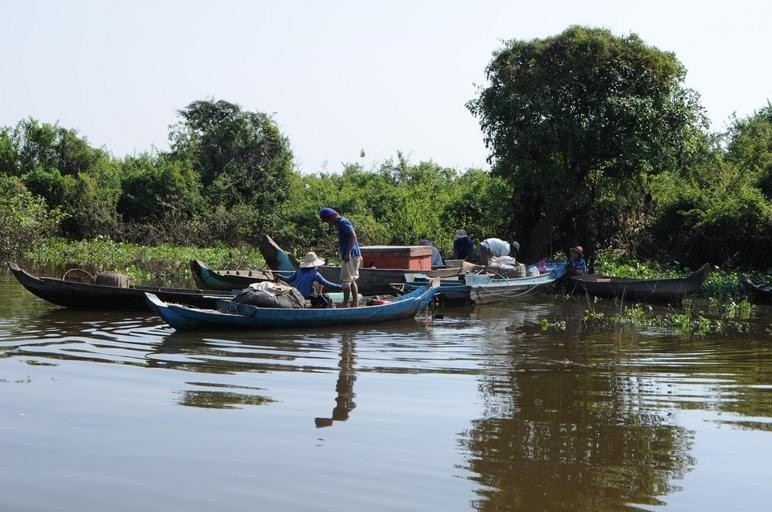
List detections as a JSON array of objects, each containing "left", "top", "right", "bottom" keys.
[{"left": 359, "top": 244, "right": 432, "bottom": 269}]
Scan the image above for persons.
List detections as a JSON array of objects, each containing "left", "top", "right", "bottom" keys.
[
  {"left": 273, "top": 252, "right": 342, "bottom": 308},
  {"left": 479, "top": 238, "right": 520, "bottom": 265},
  {"left": 568, "top": 245, "right": 586, "bottom": 275},
  {"left": 453, "top": 230, "right": 473, "bottom": 261},
  {"left": 320, "top": 208, "right": 361, "bottom": 308},
  {"left": 332, "top": 334, "right": 357, "bottom": 422},
  {"left": 419, "top": 240, "right": 442, "bottom": 265}
]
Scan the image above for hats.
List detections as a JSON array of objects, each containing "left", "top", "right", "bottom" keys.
[
  {"left": 298, "top": 251, "right": 325, "bottom": 269},
  {"left": 570, "top": 246, "right": 584, "bottom": 257},
  {"left": 511, "top": 241, "right": 520, "bottom": 253},
  {"left": 319, "top": 208, "right": 338, "bottom": 223},
  {"left": 455, "top": 230, "right": 468, "bottom": 238}
]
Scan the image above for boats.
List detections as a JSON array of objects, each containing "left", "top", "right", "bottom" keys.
[
  {"left": 187, "top": 258, "right": 273, "bottom": 292},
  {"left": 137, "top": 280, "right": 440, "bottom": 332},
  {"left": 258, "top": 233, "right": 463, "bottom": 294},
  {"left": 735, "top": 274, "right": 772, "bottom": 304},
  {"left": 393, "top": 257, "right": 568, "bottom": 304},
  {"left": 562, "top": 262, "right": 712, "bottom": 299},
  {"left": 6, "top": 259, "right": 236, "bottom": 311}
]
